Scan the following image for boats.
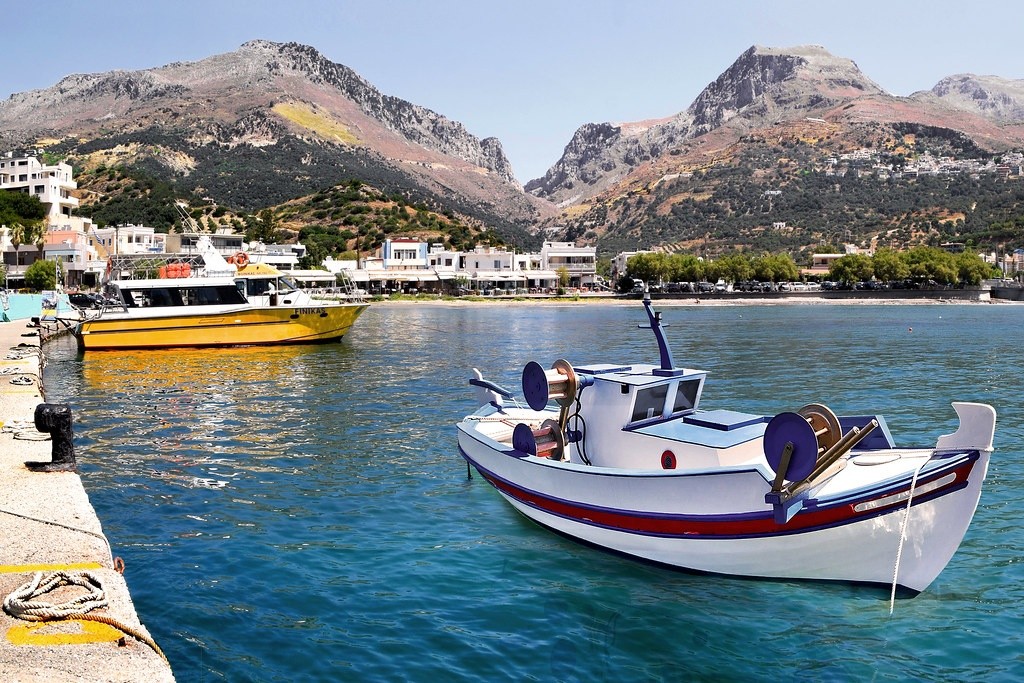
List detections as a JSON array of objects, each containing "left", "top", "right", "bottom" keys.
[
  {"left": 39, "top": 199, "right": 372, "bottom": 353},
  {"left": 455, "top": 290, "right": 996, "bottom": 599}
]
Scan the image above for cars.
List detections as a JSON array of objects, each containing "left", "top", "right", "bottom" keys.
[{"left": 631, "top": 279, "right": 913, "bottom": 293}]
